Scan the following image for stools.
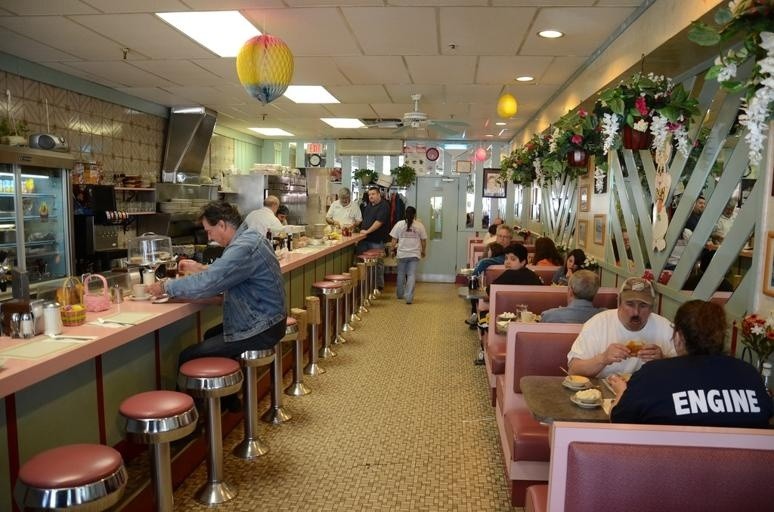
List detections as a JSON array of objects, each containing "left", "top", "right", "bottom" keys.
[
  {"left": 232, "top": 248, "right": 386, "bottom": 460},
  {"left": 115, "top": 356, "right": 244, "bottom": 511},
  {"left": 12, "top": 443, "right": 128, "bottom": 511}
]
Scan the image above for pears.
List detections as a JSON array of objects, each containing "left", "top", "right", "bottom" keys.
[{"left": 497, "top": 94, "right": 517, "bottom": 118}]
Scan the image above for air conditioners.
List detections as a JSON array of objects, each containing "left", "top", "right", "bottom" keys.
[{"left": 335, "top": 136, "right": 404, "bottom": 156}]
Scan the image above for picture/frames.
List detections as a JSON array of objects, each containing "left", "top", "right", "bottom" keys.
[
  {"left": 575, "top": 218, "right": 589, "bottom": 247},
  {"left": 481, "top": 167, "right": 508, "bottom": 198},
  {"left": 577, "top": 183, "right": 591, "bottom": 212},
  {"left": 761, "top": 230, "right": 774, "bottom": 298},
  {"left": 592, "top": 212, "right": 606, "bottom": 247},
  {"left": 456, "top": 160, "right": 471, "bottom": 174}
]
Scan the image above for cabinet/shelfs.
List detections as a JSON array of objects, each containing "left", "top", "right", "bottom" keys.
[{"left": 111, "top": 184, "right": 159, "bottom": 217}]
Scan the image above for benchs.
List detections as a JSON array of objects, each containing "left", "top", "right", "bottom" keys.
[{"left": 468, "top": 239, "right": 774, "bottom": 512}]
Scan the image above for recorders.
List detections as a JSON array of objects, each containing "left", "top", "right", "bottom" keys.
[{"left": 29, "top": 132, "right": 71, "bottom": 152}]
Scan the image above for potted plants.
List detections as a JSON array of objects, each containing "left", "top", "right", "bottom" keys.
[{"left": 351, "top": 167, "right": 377, "bottom": 188}]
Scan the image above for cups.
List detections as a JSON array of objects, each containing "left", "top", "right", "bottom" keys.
[
  {"left": 114, "top": 200, "right": 157, "bottom": 213},
  {"left": 341, "top": 222, "right": 352, "bottom": 237},
  {"left": 0, "top": 177, "right": 34, "bottom": 193},
  {"left": 132, "top": 284, "right": 148, "bottom": 297},
  {"left": 314, "top": 223, "right": 326, "bottom": 239}
]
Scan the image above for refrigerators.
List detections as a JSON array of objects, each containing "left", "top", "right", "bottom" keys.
[{"left": 0, "top": 143, "right": 76, "bottom": 298}]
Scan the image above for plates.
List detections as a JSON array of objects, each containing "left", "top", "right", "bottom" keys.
[
  {"left": 558, "top": 365, "right": 618, "bottom": 409},
  {"left": 159, "top": 197, "right": 209, "bottom": 214},
  {"left": 127, "top": 294, "right": 152, "bottom": 301},
  {"left": 495, "top": 304, "right": 542, "bottom": 328}
]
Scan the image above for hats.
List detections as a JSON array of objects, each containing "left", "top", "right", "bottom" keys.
[{"left": 618, "top": 277, "right": 655, "bottom": 307}]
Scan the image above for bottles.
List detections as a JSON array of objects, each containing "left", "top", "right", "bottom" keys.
[
  {"left": 18, "top": 312, "right": 36, "bottom": 339},
  {"left": 40, "top": 301, "right": 64, "bottom": 338},
  {"left": 111, "top": 283, "right": 122, "bottom": 304},
  {"left": 142, "top": 269, "right": 155, "bottom": 287},
  {"left": 38, "top": 199, "right": 48, "bottom": 218},
  {"left": 264, "top": 225, "right": 294, "bottom": 257},
  {"left": 8, "top": 312, "right": 22, "bottom": 339}
]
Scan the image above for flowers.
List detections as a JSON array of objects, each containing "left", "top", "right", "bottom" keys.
[
  {"left": 685, "top": 0, "right": 773, "bottom": 171},
  {"left": 506, "top": 52, "right": 703, "bottom": 186}
]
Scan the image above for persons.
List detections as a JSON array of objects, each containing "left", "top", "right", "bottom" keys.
[
  {"left": 607, "top": 297, "right": 774, "bottom": 428},
  {"left": 244, "top": 195, "right": 288, "bottom": 246},
  {"left": 464, "top": 216, "right": 564, "bottom": 266},
  {"left": 352, "top": 186, "right": 392, "bottom": 294},
  {"left": 388, "top": 206, "right": 428, "bottom": 305},
  {"left": 275, "top": 204, "right": 289, "bottom": 226},
  {"left": 550, "top": 248, "right": 587, "bottom": 287},
  {"left": 608, "top": 187, "right": 743, "bottom": 290},
  {"left": 538, "top": 268, "right": 610, "bottom": 324},
  {"left": 325, "top": 187, "right": 363, "bottom": 232},
  {"left": 565, "top": 274, "right": 679, "bottom": 379},
  {"left": 464, "top": 242, "right": 506, "bottom": 329},
  {"left": 474, "top": 243, "right": 544, "bottom": 364},
  {"left": 143, "top": 197, "right": 288, "bottom": 435}
]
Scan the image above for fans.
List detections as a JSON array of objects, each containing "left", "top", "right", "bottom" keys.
[{"left": 387, "top": 93, "right": 469, "bottom": 138}]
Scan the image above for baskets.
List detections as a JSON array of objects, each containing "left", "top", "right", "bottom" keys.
[
  {"left": 84, "top": 274, "right": 110, "bottom": 313},
  {"left": 59, "top": 300, "right": 87, "bottom": 327}
]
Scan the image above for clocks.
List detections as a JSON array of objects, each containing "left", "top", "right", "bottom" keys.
[{"left": 309, "top": 153, "right": 321, "bottom": 167}]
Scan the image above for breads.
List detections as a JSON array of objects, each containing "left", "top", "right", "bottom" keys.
[{"left": 626, "top": 341, "right": 643, "bottom": 356}]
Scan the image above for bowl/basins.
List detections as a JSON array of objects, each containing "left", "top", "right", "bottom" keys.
[{"left": 112, "top": 174, "right": 154, "bottom": 188}]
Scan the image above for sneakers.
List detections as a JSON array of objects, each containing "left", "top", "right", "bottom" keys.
[
  {"left": 464, "top": 314, "right": 478, "bottom": 327},
  {"left": 223, "top": 397, "right": 242, "bottom": 412}
]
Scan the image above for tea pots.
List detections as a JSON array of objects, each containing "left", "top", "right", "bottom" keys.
[{"left": 467, "top": 274, "right": 480, "bottom": 291}]
